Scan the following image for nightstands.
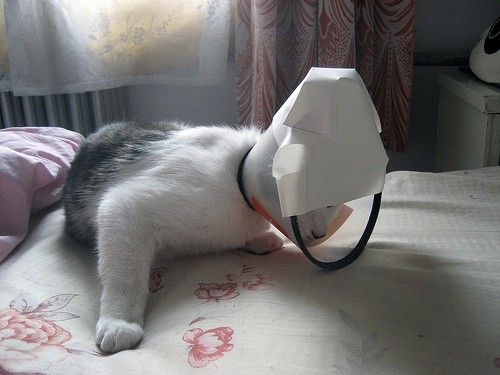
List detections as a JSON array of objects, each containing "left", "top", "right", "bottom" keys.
[{"left": 432, "top": 74, "right": 500, "bottom": 171}]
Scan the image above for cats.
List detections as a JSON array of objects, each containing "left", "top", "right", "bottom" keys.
[{"left": 61, "top": 120, "right": 334, "bottom": 353}]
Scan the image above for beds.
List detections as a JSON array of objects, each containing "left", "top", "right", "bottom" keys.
[{"left": 0, "top": 159, "right": 500, "bottom": 375}]
